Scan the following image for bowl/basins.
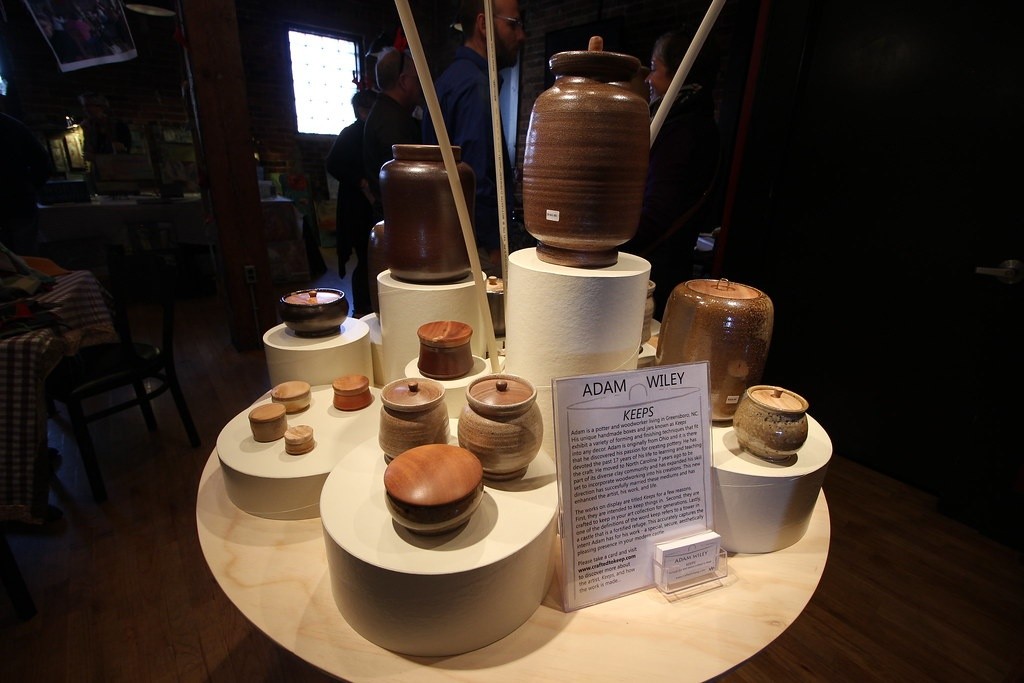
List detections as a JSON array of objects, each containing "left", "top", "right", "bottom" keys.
[
  {"left": 332, "top": 375, "right": 371, "bottom": 411},
  {"left": 271, "top": 381, "right": 312, "bottom": 413},
  {"left": 248, "top": 404, "right": 288, "bottom": 443},
  {"left": 279, "top": 289, "right": 349, "bottom": 337},
  {"left": 284, "top": 425, "right": 316, "bottom": 455},
  {"left": 384, "top": 443, "right": 483, "bottom": 533}
]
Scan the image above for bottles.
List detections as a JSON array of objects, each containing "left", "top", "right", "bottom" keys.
[{"left": 250, "top": 50, "right": 809, "bottom": 479}]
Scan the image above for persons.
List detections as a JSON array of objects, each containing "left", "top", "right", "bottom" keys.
[
  {"left": 0, "top": 110, "right": 50, "bottom": 261},
  {"left": 79, "top": 92, "right": 130, "bottom": 162},
  {"left": 327, "top": 0, "right": 525, "bottom": 320},
  {"left": 36, "top": 0, "right": 133, "bottom": 59},
  {"left": 625, "top": 25, "right": 730, "bottom": 326}
]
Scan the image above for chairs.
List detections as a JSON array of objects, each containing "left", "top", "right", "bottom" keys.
[{"left": 0, "top": 236, "right": 201, "bottom": 624}]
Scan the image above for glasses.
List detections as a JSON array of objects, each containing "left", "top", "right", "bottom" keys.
[{"left": 493, "top": 13, "right": 523, "bottom": 30}]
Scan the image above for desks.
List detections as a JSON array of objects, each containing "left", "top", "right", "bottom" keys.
[
  {"left": 36, "top": 195, "right": 213, "bottom": 247},
  {"left": 195, "top": 353, "right": 832, "bottom": 683}
]
